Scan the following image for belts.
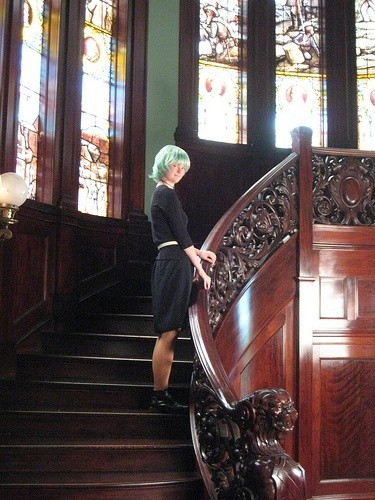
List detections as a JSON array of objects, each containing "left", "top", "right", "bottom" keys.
[{"left": 158, "top": 241, "right": 178, "bottom": 251}]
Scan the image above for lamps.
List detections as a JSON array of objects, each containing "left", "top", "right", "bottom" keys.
[{"left": 0, "top": 172, "right": 27, "bottom": 240}]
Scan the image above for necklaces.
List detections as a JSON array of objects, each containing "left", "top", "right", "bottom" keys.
[{"left": 160, "top": 178, "right": 174, "bottom": 188}]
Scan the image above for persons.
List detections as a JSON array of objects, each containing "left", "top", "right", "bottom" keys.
[{"left": 145, "top": 144, "right": 216, "bottom": 412}]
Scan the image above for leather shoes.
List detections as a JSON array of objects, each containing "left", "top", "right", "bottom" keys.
[{"left": 149, "top": 390, "right": 189, "bottom": 414}]
